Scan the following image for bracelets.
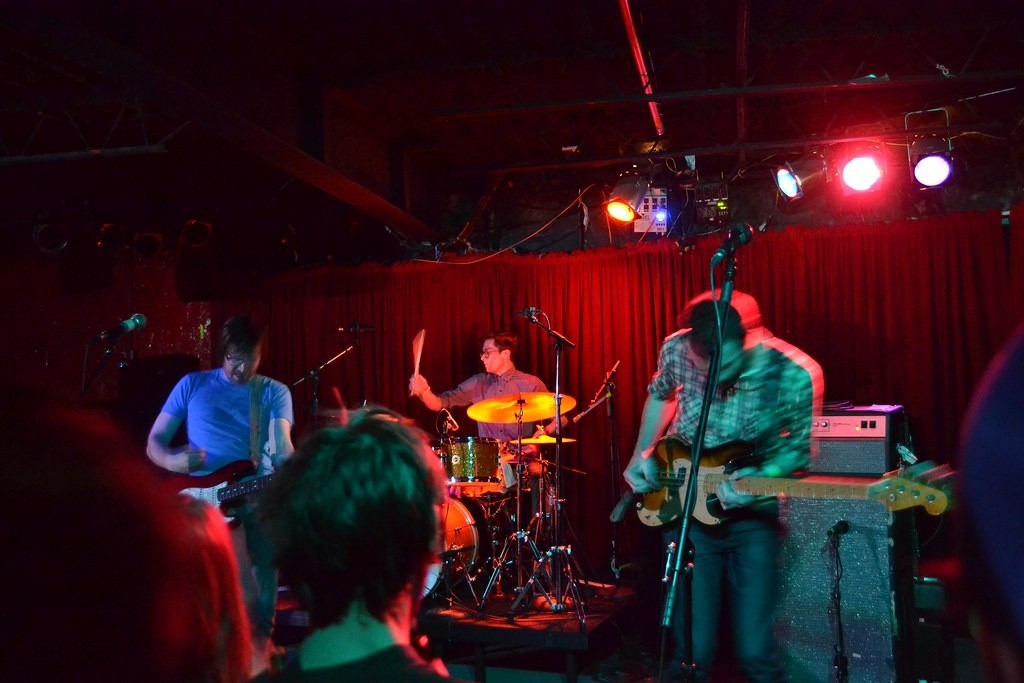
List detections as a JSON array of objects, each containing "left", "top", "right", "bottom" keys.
[{"left": 418, "top": 386, "right": 431, "bottom": 398}]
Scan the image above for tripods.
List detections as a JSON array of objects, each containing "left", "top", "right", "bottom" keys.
[{"left": 464, "top": 315, "right": 599, "bottom": 630}]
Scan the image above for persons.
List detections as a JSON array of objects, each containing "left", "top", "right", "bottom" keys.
[
  {"left": 148, "top": 315, "right": 294, "bottom": 657},
  {"left": 0, "top": 399, "right": 254, "bottom": 683},
  {"left": 957, "top": 327, "right": 1023, "bottom": 683},
  {"left": 256, "top": 409, "right": 449, "bottom": 683},
  {"left": 623, "top": 297, "right": 814, "bottom": 683},
  {"left": 409, "top": 333, "right": 568, "bottom": 587}
]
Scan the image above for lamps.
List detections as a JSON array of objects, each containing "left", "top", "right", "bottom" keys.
[{"left": 603, "top": 109, "right": 967, "bottom": 225}]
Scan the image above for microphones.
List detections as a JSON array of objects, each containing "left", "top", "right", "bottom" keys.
[
  {"left": 827, "top": 520, "right": 848, "bottom": 536},
  {"left": 518, "top": 308, "right": 537, "bottom": 316},
  {"left": 90, "top": 314, "right": 148, "bottom": 344},
  {"left": 337, "top": 322, "right": 376, "bottom": 333},
  {"left": 573, "top": 392, "right": 613, "bottom": 423},
  {"left": 711, "top": 220, "right": 755, "bottom": 264},
  {"left": 443, "top": 408, "right": 458, "bottom": 431}
]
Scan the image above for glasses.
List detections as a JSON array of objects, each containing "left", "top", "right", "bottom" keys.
[
  {"left": 225, "top": 353, "right": 254, "bottom": 365},
  {"left": 480, "top": 349, "right": 500, "bottom": 358},
  {"left": 697, "top": 355, "right": 738, "bottom": 377}
]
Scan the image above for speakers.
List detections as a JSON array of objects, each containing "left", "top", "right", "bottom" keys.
[{"left": 770, "top": 492, "right": 917, "bottom": 683}]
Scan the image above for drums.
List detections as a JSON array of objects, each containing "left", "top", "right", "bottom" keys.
[
  {"left": 417, "top": 496, "right": 475, "bottom": 600},
  {"left": 433, "top": 434, "right": 499, "bottom": 481},
  {"left": 450, "top": 466, "right": 508, "bottom": 499}
]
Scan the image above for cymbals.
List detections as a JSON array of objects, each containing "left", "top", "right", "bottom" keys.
[
  {"left": 509, "top": 433, "right": 577, "bottom": 446},
  {"left": 319, "top": 409, "right": 357, "bottom": 415},
  {"left": 467, "top": 390, "right": 575, "bottom": 424}
]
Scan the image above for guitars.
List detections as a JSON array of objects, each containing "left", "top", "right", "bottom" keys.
[
  {"left": 634, "top": 436, "right": 962, "bottom": 526},
  {"left": 151, "top": 458, "right": 286, "bottom": 528}
]
